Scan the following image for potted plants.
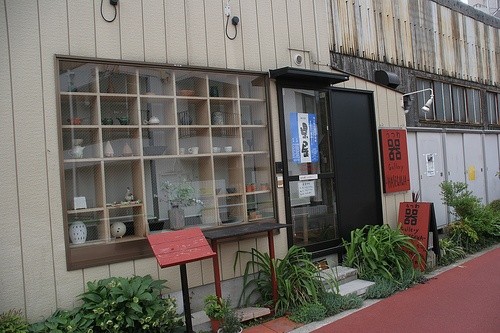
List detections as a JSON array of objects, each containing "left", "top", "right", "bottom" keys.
[{"left": 202, "top": 295, "right": 228, "bottom": 333}]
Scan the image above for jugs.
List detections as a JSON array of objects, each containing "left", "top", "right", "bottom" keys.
[
  {"left": 116, "top": 116, "right": 129, "bottom": 125},
  {"left": 69, "top": 145, "right": 85, "bottom": 159},
  {"left": 67, "top": 115, "right": 81, "bottom": 125}
]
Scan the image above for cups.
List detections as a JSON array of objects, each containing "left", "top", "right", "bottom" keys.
[
  {"left": 188, "top": 147, "right": 199, "bottom": 155},
  {"left": 179, "top": 148, "right": 185, "bottom": 155}
]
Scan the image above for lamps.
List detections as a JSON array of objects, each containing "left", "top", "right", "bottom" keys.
[{"left": 403, "top": 88, "right": 434, "bottom": 113}]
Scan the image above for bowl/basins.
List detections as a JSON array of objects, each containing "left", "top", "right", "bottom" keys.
[
  {"left": 224, "top": 147, "right": 232, "bottom": 152},
  {"left": 226, "top": 187, "right": 235, "bottom": 194},
  {"left": 177, "top": 90, "right": 194, "bottom": 96},
  {"left": 143, "top": 145, "right": 168, "bottom": 156},
  {"left": 216, "top": 188, "right": 221, "bottom": 195}
]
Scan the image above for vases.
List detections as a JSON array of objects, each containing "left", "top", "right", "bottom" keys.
[{"left": 168, "top": 205, "right": 186, "bottom": 230}]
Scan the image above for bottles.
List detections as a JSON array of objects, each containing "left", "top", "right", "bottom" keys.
[
  {"left": 210, "top": 86, "right": 219, "bottom": 97},
  {"left": 261, "top": 183, "right": 267, "bottom": 191},
  {"left": 103, "top": 118, "right": 113, "bottom": 125},
  {"left": 122, "top": 143, "right": 133, "bottom": 157},
  {"left": 104, "top": 141, "right": 114, "bottom": 157},
  {"left": 212, "top": 111, "right": 223, "bottom": 125},
  {"left": 246, "top": 183, "right": 254, "bottom": 192},
  {"left": 247, "top": 208, "right": 256, "bottom": 220}
]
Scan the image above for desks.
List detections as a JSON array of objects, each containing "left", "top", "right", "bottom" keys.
[{"left": 203, "top": 223, "right": 294, "bottom": 307}]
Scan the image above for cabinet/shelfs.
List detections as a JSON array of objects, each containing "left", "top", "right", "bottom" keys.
[{"left": 54, "top": 53, "right": 280, "bottom": 271}]
